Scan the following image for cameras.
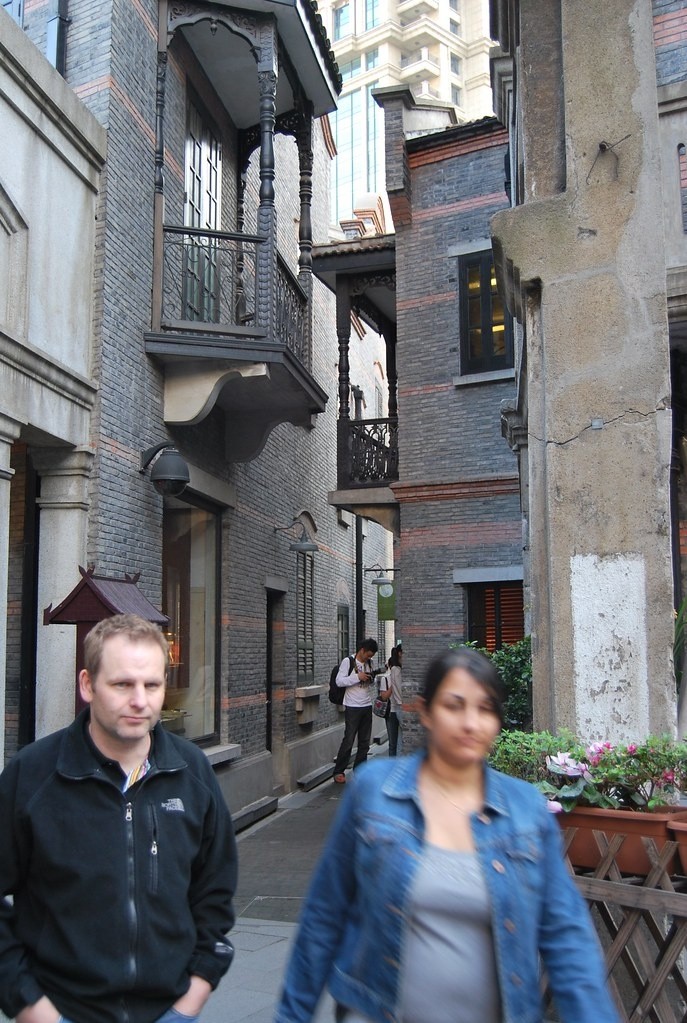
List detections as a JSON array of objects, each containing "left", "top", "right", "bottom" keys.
[{"left": 364, "top": 667, "right": 387, "bottom": 683}]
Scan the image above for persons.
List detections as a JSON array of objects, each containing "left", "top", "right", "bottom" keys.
[
  {"left": 380, "top": 644, "right": 403, "bottom": 758},
  {"left": 270, "top": 647, "right": 618, "bottom": 1023},
  {"left": 332, "top": 638, "right": 379, "bottom": 783},
  {"left": 0, "top": 614, "right": 237, "bottom": 1023}
]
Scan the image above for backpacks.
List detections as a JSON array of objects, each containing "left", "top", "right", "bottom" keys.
[{"left": 329, "top": 656, "right": 355, "bottom": 705}]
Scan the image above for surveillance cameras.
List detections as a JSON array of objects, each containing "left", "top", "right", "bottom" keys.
[{"left": 150, "top": 449, "right": 189, "bottom": 498}]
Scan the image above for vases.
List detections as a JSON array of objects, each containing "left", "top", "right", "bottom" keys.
[
  {"left": 549, "top": 798, "right": 687, "bottom": 878},
  {"left": 667, "top": 817, "right": 687, "bottom": 878}
]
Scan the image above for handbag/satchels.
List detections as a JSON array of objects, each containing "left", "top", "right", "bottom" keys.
[{"left": 374, "top": 676, "right": 390, "bottom": 718}]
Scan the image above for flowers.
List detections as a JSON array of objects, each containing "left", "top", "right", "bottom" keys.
[{"left": 532, "top": 733, "right": 687, "bottom": 813}]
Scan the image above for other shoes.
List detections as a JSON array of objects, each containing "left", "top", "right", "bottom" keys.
[{"left": 333, "top": 773, "right": 346, "bottom": 782}]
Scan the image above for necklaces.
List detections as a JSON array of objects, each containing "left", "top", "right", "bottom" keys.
[{"left": 436, "top": 786, "right": 467, "bottom": 814}]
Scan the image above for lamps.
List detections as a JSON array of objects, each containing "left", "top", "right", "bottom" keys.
[
  {"left": 274, "top": 520, "right": 319, "bottom": 554},
  {"left": 364, "top": 564, "right": 393, "bottom": 585},
  {"left": 139, "top": 439, "right": 190, "bottom": 497}
]
